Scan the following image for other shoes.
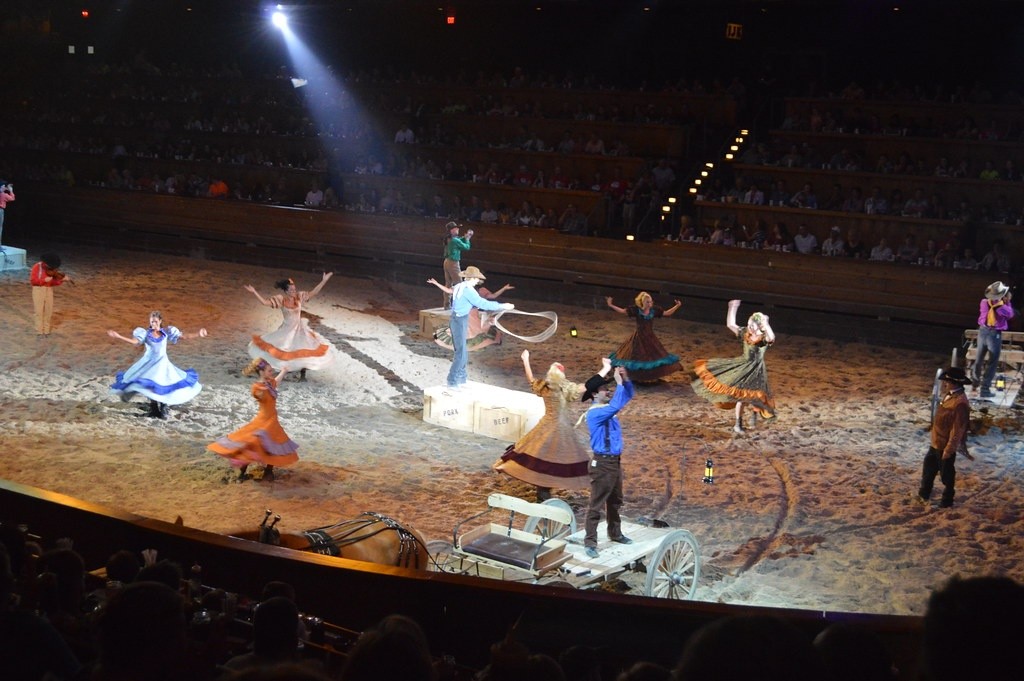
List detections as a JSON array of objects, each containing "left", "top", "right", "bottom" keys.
[
  {"left": 611, "top": 534, "right": 632, "bottom": 544},
  {"left": 584, "top": 546, "right": 600, "bottom": 558},
  {"left": 980, "top": 392, "right": 996, "bottom": 397},
  {"left": 930, "top": 503, "right": 953, "bottom": 511}
]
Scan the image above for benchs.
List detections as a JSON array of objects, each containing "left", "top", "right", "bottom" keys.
[{"left": 453, "top": 493, "right": 573, "bottom": 575}]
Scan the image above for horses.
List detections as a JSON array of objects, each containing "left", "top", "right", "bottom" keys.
[{"left": 177, "top": 511, "right": 430, "bottom": 574}]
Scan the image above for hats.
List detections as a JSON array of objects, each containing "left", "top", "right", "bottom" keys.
[
  {"left": 40, "top": 253, "right": 61, "bottom": 269},
  {"left": 458, "top": 266, "right": 486, "bottom": 279},
  {"left": 831, "top": 226, "right": 840, "bottom": 233},
  {"left": 984, "top": 281, "right": 1010, "bottom": 300},
  {"left": 938, "top": 367, "right": 972, "bottom": 385},
  {"left": 445, "top": 221, "right": 463, "bottom": 231},
  {"left": 581, "top": 374, "right": 615, "bottom": 402}
]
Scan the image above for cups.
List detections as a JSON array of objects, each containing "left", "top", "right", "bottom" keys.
[
  {"left": 106, "top": 580, "right": 120, "bottom": 589},
  {"left": 193, "top": 608, "right": 211, "bottom": 624},
  {"left": 444, "top": 655, "right": 456, "bottom": 674}
]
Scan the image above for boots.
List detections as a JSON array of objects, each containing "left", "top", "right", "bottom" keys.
[
  {"left": 147, "top": 401, "right": 159, "bottom": 418},
  {"left": 154, "top": 404, "right": 169, "bottom": 419},
  {"left": 749, "top": 411, "right": 756, "bottom": 426},
  {"left": 262, "top": 464, "right": 274, "bottom": 481},
  {"left": 733, "top": 418, "right": 746, "bottom": 434},
  {"left": 238, "top": 464, "right": 249, "bottom": 480},
  {"left": 298, "top": 368, "right": 307, "bottom": 382}
]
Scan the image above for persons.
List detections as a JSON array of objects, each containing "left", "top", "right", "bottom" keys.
[
  {"left": 912, "top": 367, "right": 972, "bottom": 508},
  {"left": 493, "top": 348, "right": 612, "bottom": 503},
  {"left": 442, "top": 221, "right": 474, "bottom": 309},
  {"left": 207, "top": 357, "right": 299, "bottom": 483},
  {"left": 963, "top": 280, "right": 1011, "bottom": 398},
  {"left": 244, "top": 270, "right": 334, "bottom": 382},
  {"left": 30, "top": 253, "right": 64, "bottom": 339},
  {"left": 607, "top": 292, "right": 684, "bottom": 384},
  {"left": 107, "top": 311, "right": 207, "bottom": 419},
  {"left": 581, "top": 365, "right": 635, "bottom": 559},
  {"left": 0, "top": 59, "right": 1024, "bottom": 282},
  {"left": 1, "top": 518, "right": 1024, "bottom": 681},
  {"left": 426, "top": 277, "right": 515, "bottom": 352},
  {"left": 427, "top": 266, "right": 515, "bottom": 386},
  {"left": 688, "top": 299, "right": 776, "bottom": 434}
]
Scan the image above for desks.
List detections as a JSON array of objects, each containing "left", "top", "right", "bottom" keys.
[
  {"left": 92, "top": 563, "right": 364, "bottom": 670},
  {"left": 1, "top": 65, "right": 1024, "bottom": 327}
]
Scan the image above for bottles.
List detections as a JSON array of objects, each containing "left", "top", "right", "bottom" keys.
[{"left": 191, "top": 561, "right": 202, "bottom": 589}]
